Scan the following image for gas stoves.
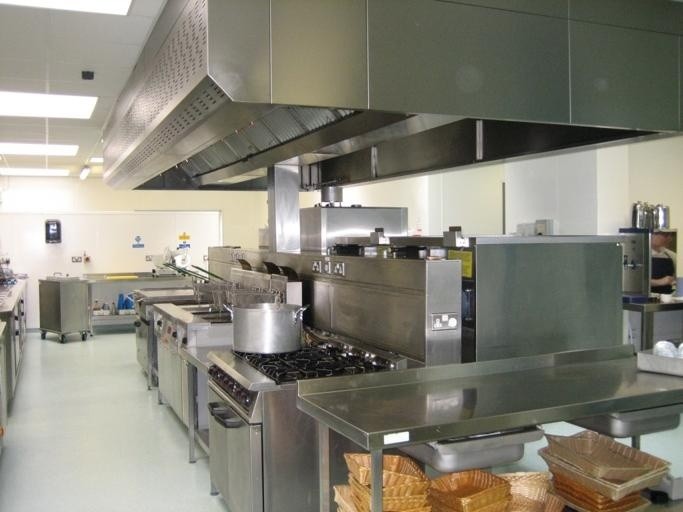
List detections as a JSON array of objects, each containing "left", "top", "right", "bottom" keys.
[{"left": 229, "top": 337, "right": 393, "bottom": 385}]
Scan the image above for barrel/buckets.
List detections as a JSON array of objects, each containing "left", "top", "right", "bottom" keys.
[{"left": 633, "top": 202, "right": 670, "bottom": 228}]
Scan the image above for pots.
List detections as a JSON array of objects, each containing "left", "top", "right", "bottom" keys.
[
  {"left": 222, "top": 302, "right": 310, "bottom": 356},
  {"left": 193, "top": 280, "right": 281, "bottom": 312}
]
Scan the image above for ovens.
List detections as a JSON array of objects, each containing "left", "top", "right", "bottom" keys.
[{"left": 206, "top": 364, "right": 265, "bottom": 512}]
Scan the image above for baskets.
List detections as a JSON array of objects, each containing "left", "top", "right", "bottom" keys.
[{"left": 333, "top": 429, "right": 674, "bottom": 510}]
